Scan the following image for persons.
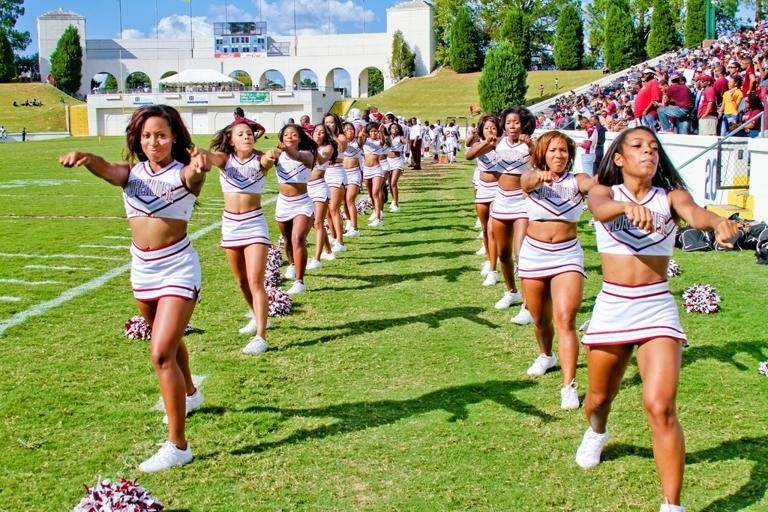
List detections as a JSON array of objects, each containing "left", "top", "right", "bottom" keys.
[
  {"left": 533, "top": 77, "right": 605, "bottom": 177},
  {"left": 599, "top": 20, "right": 768, "bottom": 137},
  {"left": 571, "top": 123, "right": 746, "bottom": 512},
  {"left": 519, "top": 132, "right": 602, "bottom": 410},
  {"left": 272, "top": 105, "right": 461, "bottom": 296},
  {"left": 193, "top": 119, "right": 282, "bottom": 359},
  {"left": 142, "top": 84, "right": 150, "bottom": 93},
  {"left": 56, "top": 103, "right": 215, "bottom": 475},
  {"left": 0, "top": 125, "right": 8, "bottom": 139},
  {"left": 293, "top": 83, "right": 298, "bottom": 90},
  {"left": 223, "top": 106, "right": 265, "bottom": 142},
  {"left": 21, "top": 127, "right": 27, "bottom": 142},
  {"left": 185, "top": 83, "right": 262, "bottom": 93},
  {"left": 464, "top": 105, "right": 537, "bottom": 322},
  {"left": 11, "top": 98, "right": 43, "bottom": 108},
  {"left": 92, "top": 84, "right": 101, "bottom": 95},
  {"left": 465, "top": 113, "right": 503, "bottom": 286}
]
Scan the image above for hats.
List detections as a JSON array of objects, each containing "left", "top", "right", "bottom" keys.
[
  {"left": 642, "top": 69, "right": 657, "bottom": 75},
  {"left": 693, "top": 73, "right": 712, "bottom": 84}
]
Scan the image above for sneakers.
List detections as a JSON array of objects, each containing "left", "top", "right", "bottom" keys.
[
  {"left": 240, "top": 333, "right": 270, "bottom": 355},
  {"left": 496, "top": 288, "right": 522, "bottom": 311},
  {"left": 576, "top": 426, "right": 610, "bottom": 469},
  {"left": 509, "top": 302, "right": 536, "bottom": 325},
  {"left": 527, "top": 352, "right": 557, "bottom": 377},
  {"left": 560, "top": 385, "right": 580, "bottom": 410},
  {"left": 306, "top": 220, "right": 360, "bottom": 271},
  {"left": 367, "top": 202, "right": 400, "bottom": 226},
  {"left": 139, "top": 440, "right": 193, "bottom": 473},
  {"left": 238, "top": 314, "right": 271, "bottom": 335},
  {"left": 284, "top": 263, "right": 306, "bottom": 295},
  {"left": 475, "top": 217, "right": 503, "bottom": 287},
  {"left": 657, "top": 502, "right": 686, "bottom": 511},
  {"left": 163, "top": 391, "right": 205, "bottom": 423}
]
